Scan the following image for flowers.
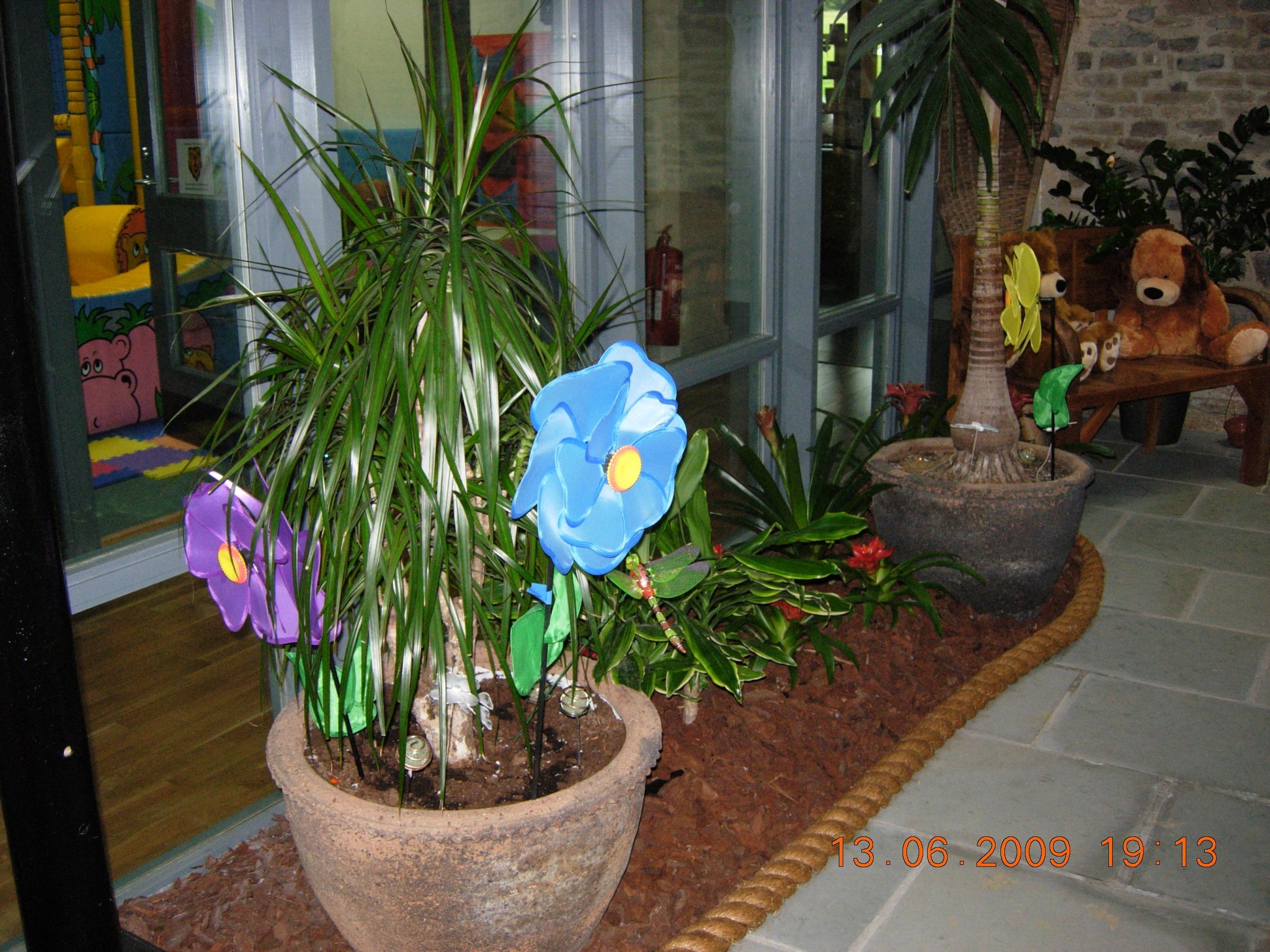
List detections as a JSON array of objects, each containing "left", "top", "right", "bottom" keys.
[
  {"left": 1001, "top": 242, "right": 1085, "bottom": 480},
  {"left": 182, "top": 457, "right": 367, "bottom": 785},
  {"left": 507, "top": 341, "right": 687, "bottom": 791}
]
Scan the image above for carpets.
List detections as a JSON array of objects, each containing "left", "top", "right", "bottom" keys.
[{"left": 87, "top": 422, "right": 219, "bottom": 489}]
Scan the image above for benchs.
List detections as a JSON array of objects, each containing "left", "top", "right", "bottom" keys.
[{"left": 948, "top": 230, "right": 1270, "bottom": 486}]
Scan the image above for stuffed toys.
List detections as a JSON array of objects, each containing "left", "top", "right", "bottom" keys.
[
  {"left": 1118, "top": 229, "right": 1269, "bottom": 368},
  {"left": 1019, "top": 227, "right": 1124, "bottom": 381}
]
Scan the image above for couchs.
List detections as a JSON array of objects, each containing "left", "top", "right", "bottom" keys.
[{"left": 64, "top": 205, "right": 242, "bottom": 435}]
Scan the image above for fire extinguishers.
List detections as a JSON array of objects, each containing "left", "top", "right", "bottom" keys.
[{"left": 644, "top": 223, "right": 684, "bottom": 346}]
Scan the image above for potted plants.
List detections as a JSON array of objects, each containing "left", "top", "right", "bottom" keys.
[
  {"left": 1035, "top": 105, "right": 1270, "bottom": 446},
  {"left": 161, "top": 0, "right": 675, "bottom": 952},
  {"left": 814, "top": 0, "right": 1095, "bottom": 614}
]
